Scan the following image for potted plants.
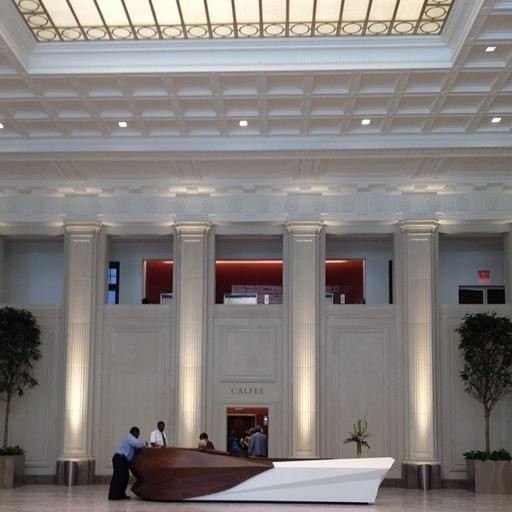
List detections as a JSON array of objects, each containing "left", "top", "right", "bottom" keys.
[
  {"left": 456, "top": 312, "right": 511, "bottom": 493},
  {"left": 1, "top": 306, "right": 44, "bottom": 487}
]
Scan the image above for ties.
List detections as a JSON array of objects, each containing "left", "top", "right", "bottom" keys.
[{"left": 162, "top": 433, "right": 166, "bottom": 447}]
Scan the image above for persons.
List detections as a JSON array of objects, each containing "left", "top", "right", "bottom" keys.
[
  {"left": 198, "top": 433, "right": 214, "bottom": 449},
  {"left": 229, "top": 425, "right": 267, "bottom": 457},
  {"left": 150, "top": 421, "right": 168, "bottom": 448},
  {"left": 109, "top": 426, "right": 148, "bottom": 500}
]
